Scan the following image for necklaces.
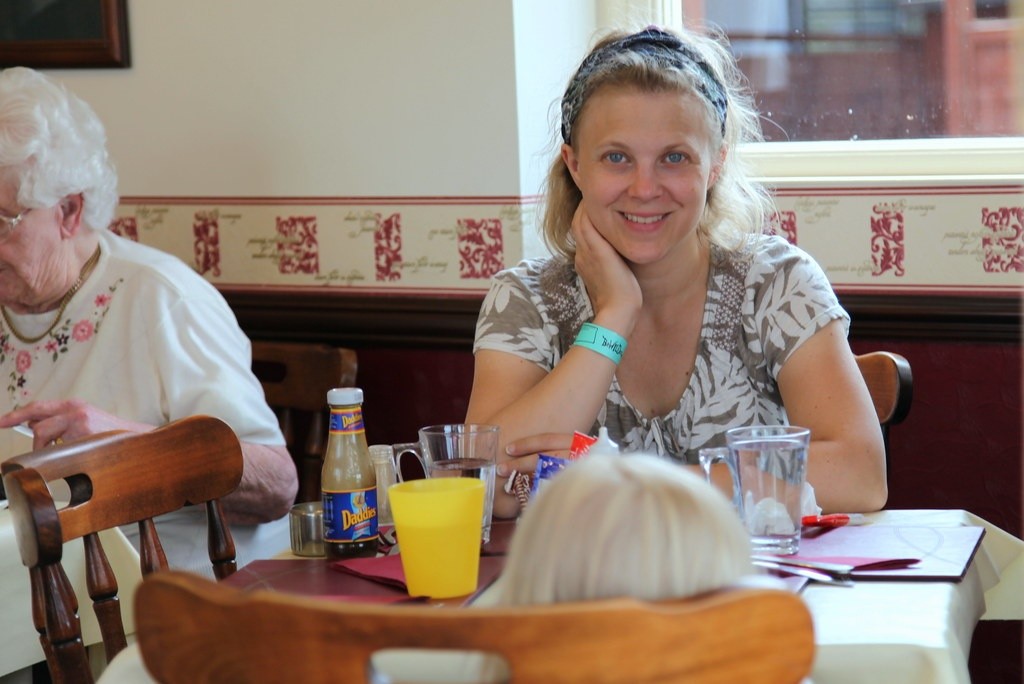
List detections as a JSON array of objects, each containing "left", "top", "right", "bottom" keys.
[{"left": 0, "top": 247, "right": 100, "bottom": 344}]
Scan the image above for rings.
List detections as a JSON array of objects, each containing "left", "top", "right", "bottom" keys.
[{"left": 50, "top": 438, "right": 63, "bottom": 446}]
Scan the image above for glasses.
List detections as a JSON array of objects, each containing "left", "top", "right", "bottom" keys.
[{"left": 0, "top": 209, "right": 32, "bottom": 243}]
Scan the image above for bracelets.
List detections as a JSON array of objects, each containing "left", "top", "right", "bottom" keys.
[{"left": 573, "top": 322, "right": 628, "bottom": 366}]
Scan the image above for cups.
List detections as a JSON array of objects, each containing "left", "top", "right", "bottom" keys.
[
  {"left": 290, "top": 503, "right": 324, "bottom": 557},
  {"left": 698, "top": 425, "right": 810, "bottom": 555},
  {"left": 390, "top": 423, "right": 500, "bottom": 544},
  {"left": 388, "top": 476, "right": 485, "bottom": 598}
]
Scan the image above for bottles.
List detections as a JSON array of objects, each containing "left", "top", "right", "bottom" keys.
[
  {"left": 320, "top": 387, "right": 379, "bottom": 559},
  {"left": 367, "top": 443, "right": 396, "bottom": 525}
]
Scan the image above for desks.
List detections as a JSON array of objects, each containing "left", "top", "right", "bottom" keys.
[
  {"left": 96, "top": 507, "right": 1022, "bottom": 683},
  {"left": 0, "top": 498, "right": 143, "bottom": 678}
]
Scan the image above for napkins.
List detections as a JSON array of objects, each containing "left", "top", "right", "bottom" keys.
[{"left": 331, "top": 554, "right": 423, "bottom": 589}]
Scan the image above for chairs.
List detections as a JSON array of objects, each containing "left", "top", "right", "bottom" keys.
[
  {"left": 854, "top": 350, "right": 913, "bottom": 442},
  {"left": 132, "top": 570, "right": 817, "bottom": 684},
  {"left": 0, "top": 415, "right": 244, "bottom": 684},
  {"left": 251, "top": 342, "right": 358, "bottom": 545}
]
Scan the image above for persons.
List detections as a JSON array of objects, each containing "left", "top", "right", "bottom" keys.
[
  {"left": 0, "top": 67, "right": 298, "bottom": 580},
  {"left": 464, "top": 23, "right": 888, "bottom": 520}
]
